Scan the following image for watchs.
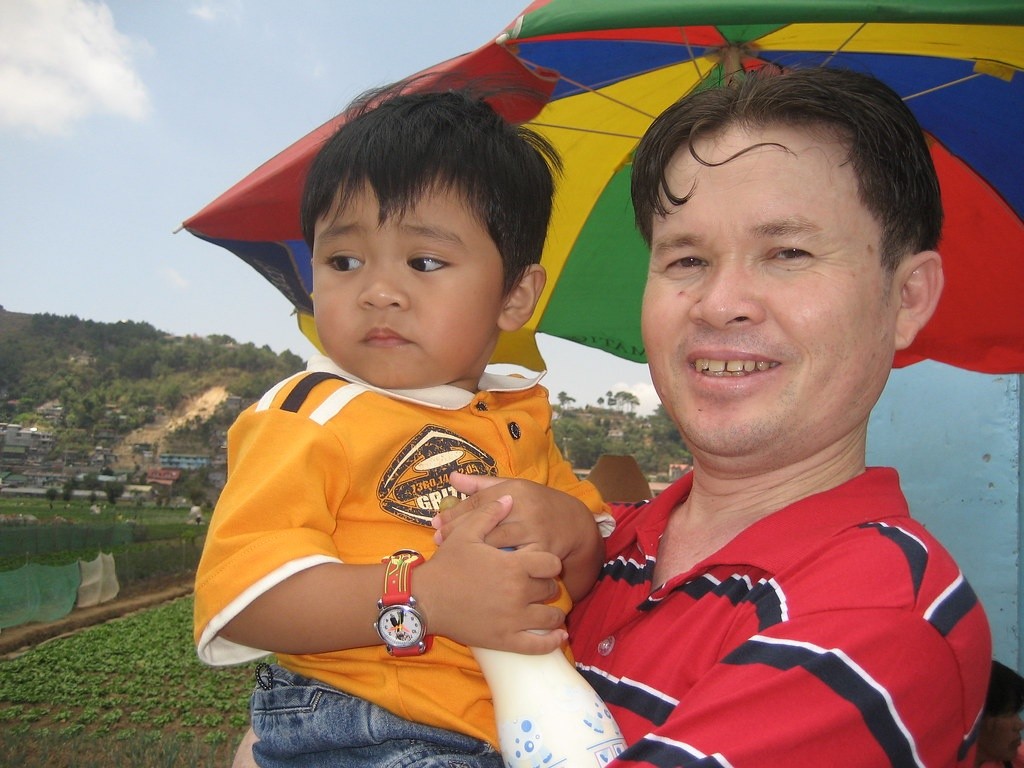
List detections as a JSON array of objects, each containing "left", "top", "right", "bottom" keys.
[{"left": 372, "top": 549, "right": 433, "bottom": 656}]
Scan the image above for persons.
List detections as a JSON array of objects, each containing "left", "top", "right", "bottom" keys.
[
  {"left": 194, "top": 73, "right": 615, "bottom": 768},
  {"left": 230, "top": 64, "right": 991, "bottom": 768}
]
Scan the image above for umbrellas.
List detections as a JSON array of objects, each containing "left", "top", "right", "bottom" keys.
[{"left": 171, "top": 0, "right": 1023, "bottom": 375}]
